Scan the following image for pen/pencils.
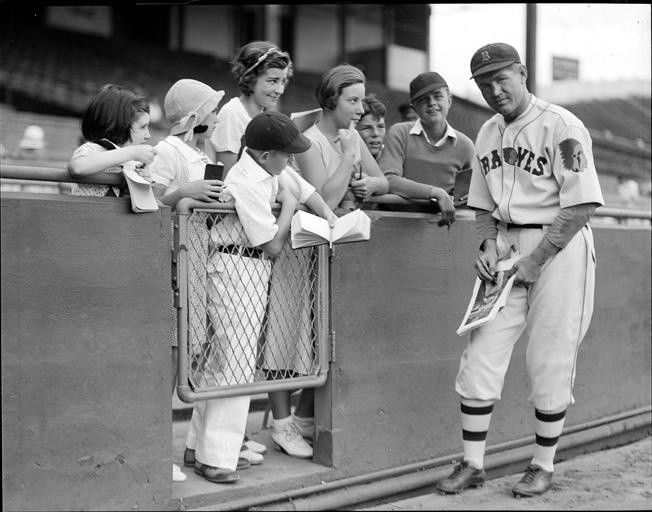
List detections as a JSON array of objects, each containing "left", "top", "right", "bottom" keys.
[
  {"left": 353, "top": 163, "right": 362, "bottom": 180},
  {"left": 478, "top": 256, "right": 498, "bottom": 281}
]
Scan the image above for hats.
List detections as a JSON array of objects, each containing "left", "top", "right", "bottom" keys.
[
  {"left": 164, "top": 78, "right": 227, "bottom": 136},
  {"left": 409, "top": 72, "right": 447, "bottom": 103},
  {"left": 245, "top": 111, "right": 313, "bottom": 154},
  {"left": 470, "top": 43, "right": 521, "bottom": 80}
]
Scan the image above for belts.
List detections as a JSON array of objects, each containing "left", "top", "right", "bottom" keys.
[
  {"left": 219, "top": 244, "right": 262, "bottom": 258},
  {"left": 507, "top": 223, "right": 543, "bottom": 228}
]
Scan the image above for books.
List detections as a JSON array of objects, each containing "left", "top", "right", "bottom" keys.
[
  {"left": 204, "top": 160, "right": 225, "bottom": 204},
  {"left": 456, "top": 256, "right": 517, "bottom": 336},
  {"left": 289, "top": 106, "right": 323, "bottom": 135},
  {"left": 121, "top": 160, "right": 159, "bottom": 214},
  {"left": 453, "top": 169, "right": 473, "bottom": 207},
  {"left": 289, "top": 208, "right": 372, "bottom": 251}
]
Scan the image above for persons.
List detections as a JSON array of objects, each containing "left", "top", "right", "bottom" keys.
[
  {"left": 68, "top": 85, "right": 158, "bottom": 197},
  {"left": 183, "top": 112, "right": 339, "bottom": 484},
  {"left": 356, "top": 97, "right": 387, "bottom": 162},
  {"left": 204, "top": 41, "right": 294, "bottom": 465},
  {"left": 397, "top": 103, "right": 420, "bottom": 122},
  {"left": 377, "top": 71, "right": 475, "bottom": 227},
  {"left": 254, "top": 64, "right": 391, "bottom": 460},
  {"left": 147, "top": 77, "right": 226, "bottom": 481},
  {"left": 436, "top": 42, "right": 605, "bottom": 498}
]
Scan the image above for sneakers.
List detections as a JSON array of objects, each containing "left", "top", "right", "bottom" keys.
[
  {"left": 435, "top": 460, "right": 487, "bottom": 494},
  {"left": 512, "top": 463, "right": 554, "bottom": 496},
  {"left": 172, "top": 417, "right": 316, "bottom": 482}
]
